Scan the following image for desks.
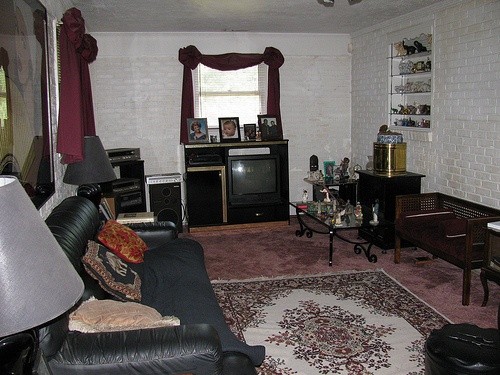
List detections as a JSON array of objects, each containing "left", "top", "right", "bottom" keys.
[{"left": 305, "top": 176, "right": 357, "bottom": 205}]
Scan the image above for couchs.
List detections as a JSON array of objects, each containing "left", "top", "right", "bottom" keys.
[{"left": 1, "top": 198, "right": 267, "bottom": 375}]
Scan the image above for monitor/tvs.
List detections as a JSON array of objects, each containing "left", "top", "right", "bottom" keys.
[{"left": 227, "top": 154, "right": 281, "bottom": 204}]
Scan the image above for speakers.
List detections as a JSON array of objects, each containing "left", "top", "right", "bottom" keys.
[{"left": 150, "top": 182, "right": 183, "bottom": 232}]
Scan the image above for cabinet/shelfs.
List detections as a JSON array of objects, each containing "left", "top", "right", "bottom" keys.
[
  {"left": 353, "top": 168, "right": 427, "bottom": 249},
  {"left": 383, "top": 35, "right": 433, "bottom": 132}
]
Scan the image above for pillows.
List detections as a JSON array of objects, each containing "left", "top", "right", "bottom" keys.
[
  {"left": 82, "top": 239, "right": 143, "bottom": 302},
  {"left": 99, "top": 220, "right": 146, "bottom": 263},
  {"left": 70, "top": 297, "right": 180, "bottom": 333}
]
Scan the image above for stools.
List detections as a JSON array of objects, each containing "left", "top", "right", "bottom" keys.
[{"left": 425, "top": 321, "right": 500, "bottom": 375}]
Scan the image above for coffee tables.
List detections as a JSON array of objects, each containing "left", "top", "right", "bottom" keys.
[{"left": 290, "top": 201, "right": 379, "bottom": 266}]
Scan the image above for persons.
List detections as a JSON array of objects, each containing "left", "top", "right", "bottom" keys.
[
  {"left": 262, "top": 119, "right": 278, "bottom": 136},
  {"left": 222, "top": 121, "right": 238, "bottom": 138},
  {"left": 211, "top": 136, "right": 217, "bottom": 143},
  {"left": 190, "top": 122, "right": 206, "bottom": 141},
  {"left": 300, "top": 189, "right": 378, "bottom": 226},
  {"left": 246, "top": 127, "right": 255, "bottom": 136}
]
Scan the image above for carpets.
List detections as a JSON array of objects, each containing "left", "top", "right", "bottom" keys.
[{"left": 208, "top": 269, "right": 454, "bottom": 375}]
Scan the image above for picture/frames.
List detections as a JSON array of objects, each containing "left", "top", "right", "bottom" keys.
[
  {"left": 257, "top": 114, "right": 283, "bottom": 140},
  {"left": 186, "top": 117, "right": 211, "bottom": 144},
  {"left": 245, "top": 125, "right": 257, "bottom": 142},
  {"left": 219, "top": 117, "right": 241, "bottom": 143}
]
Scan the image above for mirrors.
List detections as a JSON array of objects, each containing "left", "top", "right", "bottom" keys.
[{"left": 0, "top": 1, "right": 58, "bottom": 210}]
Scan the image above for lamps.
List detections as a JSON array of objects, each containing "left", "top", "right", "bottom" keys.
[
  {"left": 0, "top": 176, "right": 85, "bottom": 340},
  {"left": 66, "top": 133, "right": 116, "bottom": 186}
]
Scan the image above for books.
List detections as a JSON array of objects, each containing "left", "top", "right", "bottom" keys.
[
  {"left": 488, "top": 221, "right": 500, "bottom": 230},
  {"left": 116, "top": 212, "right": 154, "bottom": 224}
]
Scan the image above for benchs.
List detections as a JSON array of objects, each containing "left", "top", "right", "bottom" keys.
[{"left": 393, "top": 192, "right": 500, "bottom": 306}]
